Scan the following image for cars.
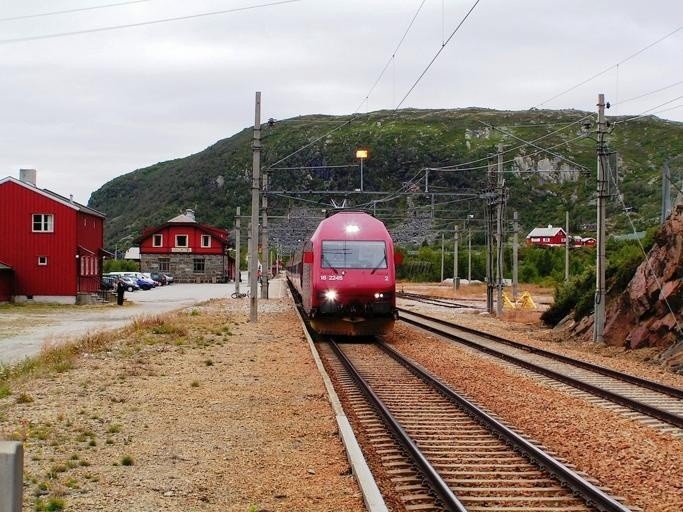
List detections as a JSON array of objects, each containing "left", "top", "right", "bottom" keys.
[{"left": 101, "top": 270, "right": 174, "bottom": 293}]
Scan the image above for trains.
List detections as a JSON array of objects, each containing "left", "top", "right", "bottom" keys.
[{"left": 285, "top": 195, "right": 399, "bottom": 339}]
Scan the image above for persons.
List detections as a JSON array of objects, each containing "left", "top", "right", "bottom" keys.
[{"left": 116, "top": 278, "right": 126, "bottom": 305}]
